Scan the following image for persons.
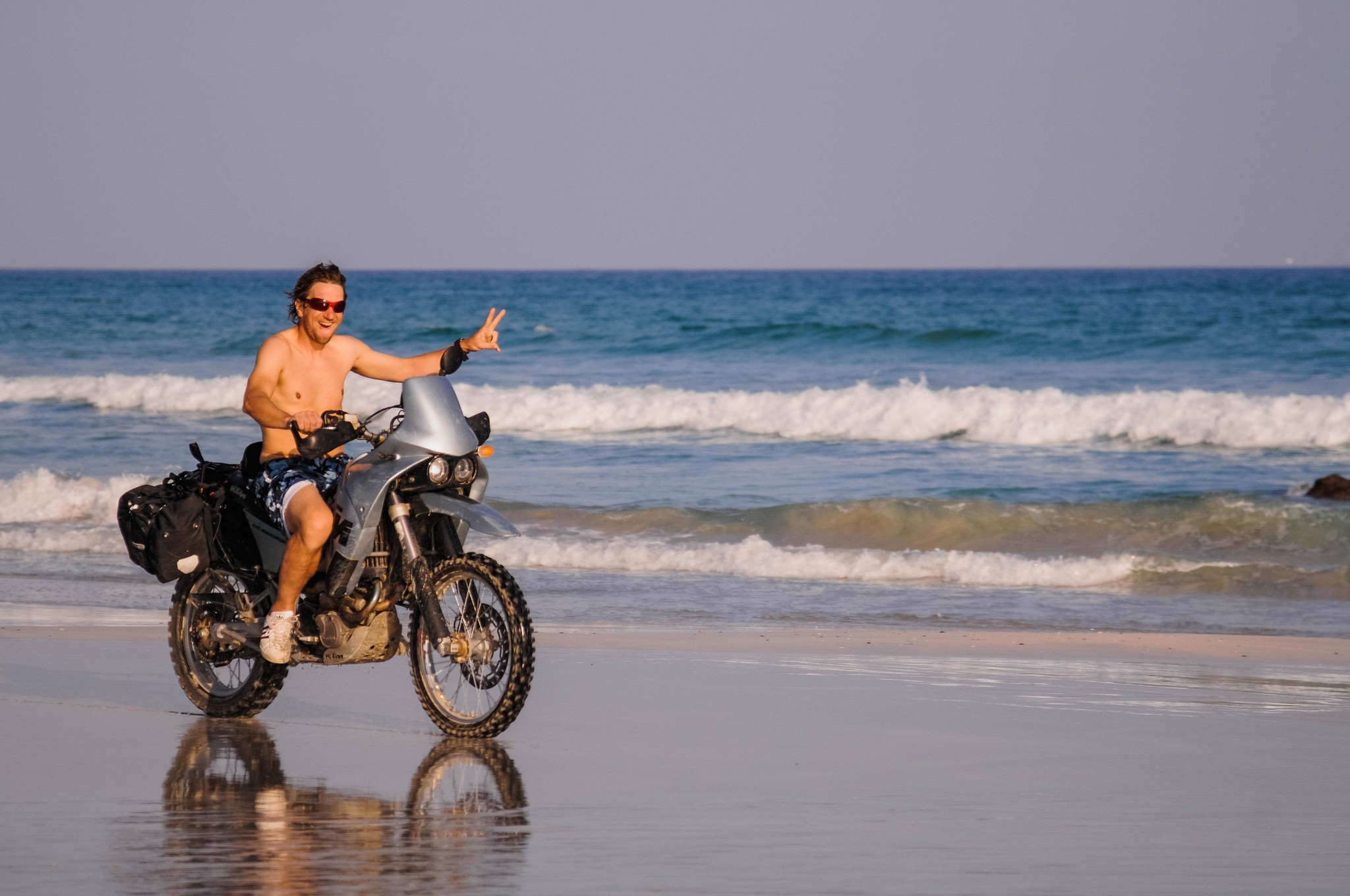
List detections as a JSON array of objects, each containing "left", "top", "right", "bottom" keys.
[{"left": 242, "top": 263, "right": 506, "bottom": 665}]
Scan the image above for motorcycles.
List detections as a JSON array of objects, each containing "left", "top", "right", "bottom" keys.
[{"left": 116, "top": 347, "right": 536, "bottom": 740}]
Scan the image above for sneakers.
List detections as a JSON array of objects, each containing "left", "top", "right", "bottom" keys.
[{"left": 259, "top": 611, "right": 300, "bottom": 664}]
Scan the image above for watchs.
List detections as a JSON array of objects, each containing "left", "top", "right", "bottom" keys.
[{"left": 452, "top": 337, "right": 469, "bottom": 362}]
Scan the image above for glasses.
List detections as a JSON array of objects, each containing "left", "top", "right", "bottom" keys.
[{"left": 300, "top": 297, "right": 346, "bottom": 313}]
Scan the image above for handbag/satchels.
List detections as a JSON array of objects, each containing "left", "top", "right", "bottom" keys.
[{"left": 117, "top": 475, "right": 211, "bottom": 584}]
